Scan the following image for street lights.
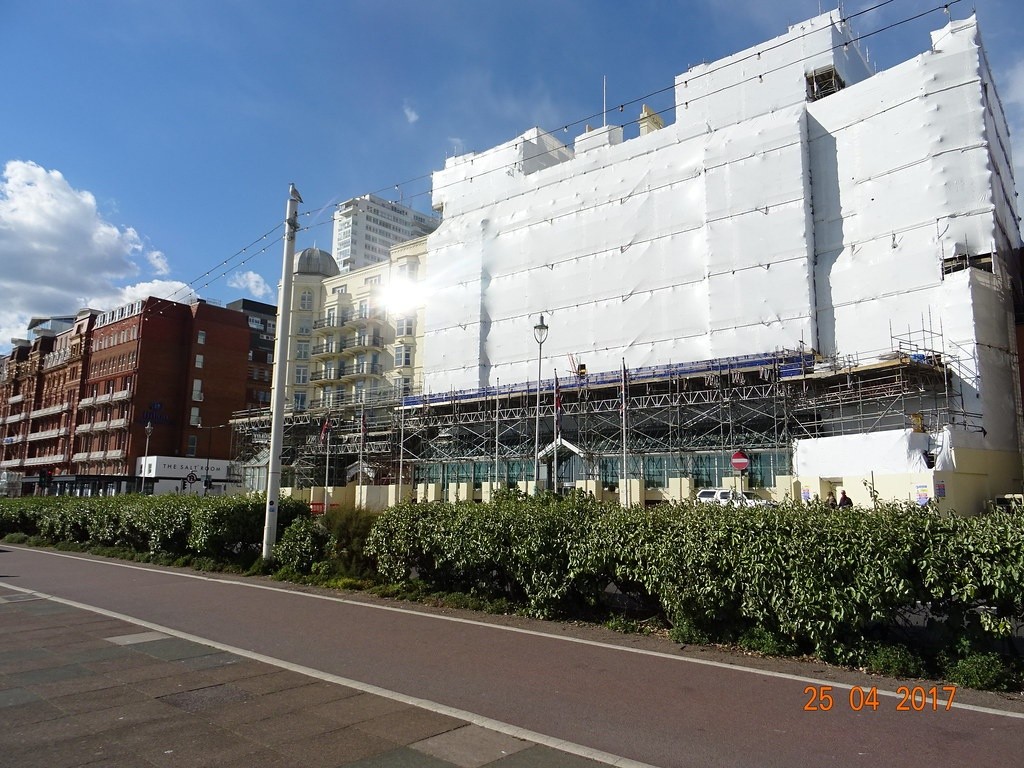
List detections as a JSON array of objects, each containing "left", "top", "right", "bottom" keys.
[
  {"left": 533, "top": 315, "right": 549, "bottom": 495},
  {"left": 141, "top": 421, "right": 155, "bottom": 492}
]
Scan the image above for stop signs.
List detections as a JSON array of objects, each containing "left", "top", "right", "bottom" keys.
[{"left": 730, "top": 452, "right": 749, "bottom": 471}]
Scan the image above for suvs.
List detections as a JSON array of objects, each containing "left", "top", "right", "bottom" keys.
[{"left": 692, "top": 488, "right": 778, "bottom": 511}]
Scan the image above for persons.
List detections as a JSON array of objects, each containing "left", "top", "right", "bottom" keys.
[
  {"left": 839, "top": 490, "right": 853, "bottom": 506},
  {"left": 589, "top": 490, "right": 595, "bottom": 500},
  {"left": 826, "top": 492, "right": 837, "bottom": 505}
]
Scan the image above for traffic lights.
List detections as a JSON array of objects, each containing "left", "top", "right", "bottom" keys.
[
  {"left": 38, "top": 469, "right": 45, "bottom": 487},
  {"left": 47, "top": 469, "right": 53, "bottom": 486}
]
